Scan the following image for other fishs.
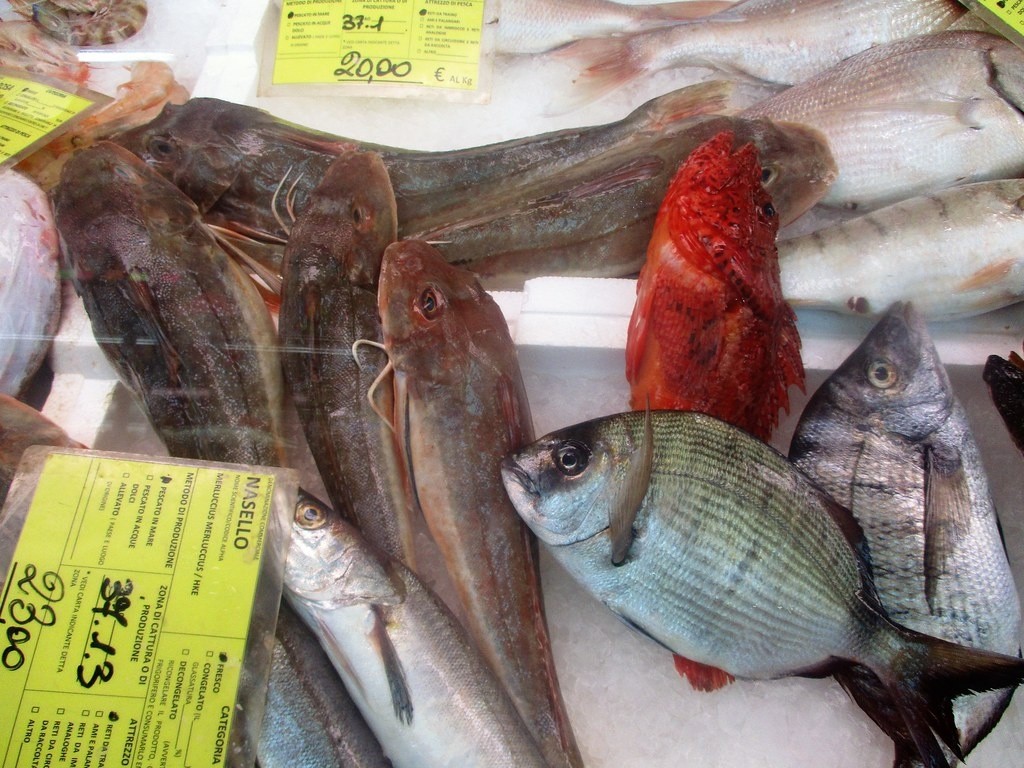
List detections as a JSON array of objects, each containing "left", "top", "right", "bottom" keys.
[{"left": 57, "top": 0, "right": 1023, "bottom": 768}]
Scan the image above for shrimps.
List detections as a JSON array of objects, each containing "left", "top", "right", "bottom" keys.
[{"left": 30, "top": 0, "right": 149, "bottom": 47}]
